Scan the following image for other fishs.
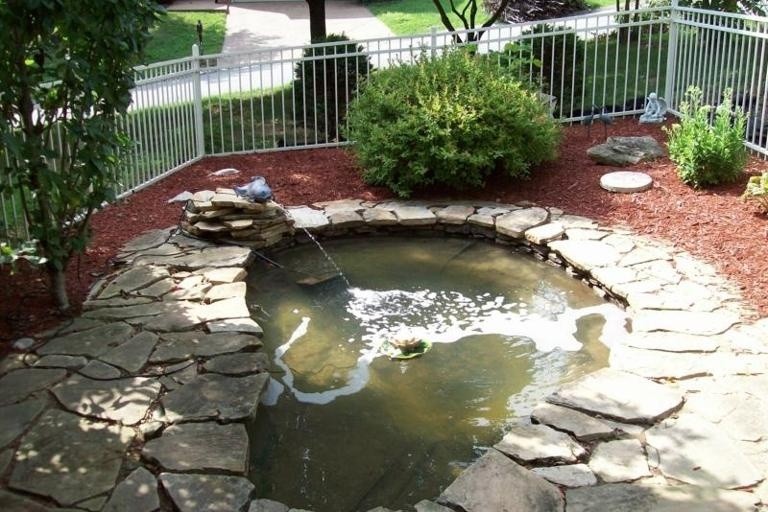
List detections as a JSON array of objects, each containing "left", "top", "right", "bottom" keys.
[{"left": 232, "top": 176, "right": 272, "bottom": 202}]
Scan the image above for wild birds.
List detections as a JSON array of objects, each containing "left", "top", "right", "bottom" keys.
[
  {"left": 599, "top": 106, "right": 617, "bottom": 140},
  {"left": 581, "top": 104, "right": 599, "bottom": 140}
]
